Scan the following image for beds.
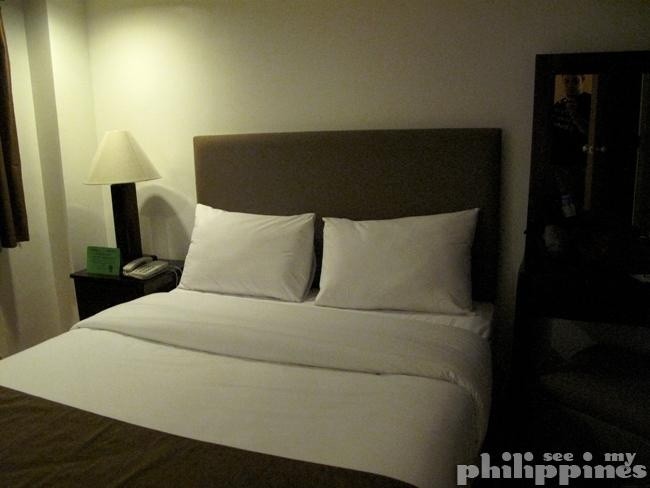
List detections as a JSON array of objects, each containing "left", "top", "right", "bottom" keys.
[{"left": 0, "top": 291, "right": 492, "bottom": 484}]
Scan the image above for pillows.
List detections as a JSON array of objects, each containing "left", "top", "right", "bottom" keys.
[{"left": 175, "top": 202, "right": 479, "bottom": 311}]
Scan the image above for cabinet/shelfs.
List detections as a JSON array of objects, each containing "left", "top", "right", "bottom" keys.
[{"left": 512, "top": 49, "right": 649, "bottom": 473}]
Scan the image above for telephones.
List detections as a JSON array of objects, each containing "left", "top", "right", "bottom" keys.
[{"left": 122, "top": 256, "right": 168, "bottom": 280}]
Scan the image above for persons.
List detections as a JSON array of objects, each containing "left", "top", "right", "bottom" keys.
[{"left": 551, "top": 69, "right": 591, "bottom": 189}]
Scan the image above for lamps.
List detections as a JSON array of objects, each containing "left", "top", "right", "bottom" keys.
[{"left": 83, "top": 133, "right": 161, "bottom": 262}]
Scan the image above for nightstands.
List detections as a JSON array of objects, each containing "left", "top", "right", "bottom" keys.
[{"left": 67, "top": 258, "right": 182, "bottom": 319}]
